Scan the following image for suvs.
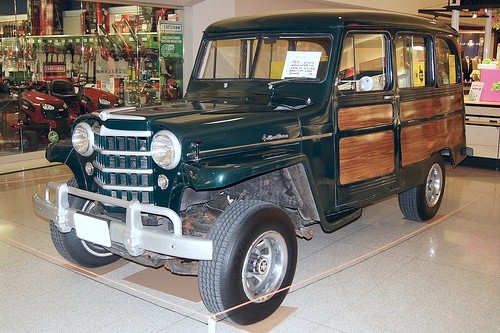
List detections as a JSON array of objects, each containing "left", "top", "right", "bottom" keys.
[{"left": 30, "top": 9, "right": 474, "bottom": 326}]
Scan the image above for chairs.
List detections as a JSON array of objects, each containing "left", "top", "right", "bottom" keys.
[
  {"left": 50, "top": 80, "right": 78, "bottom": 100},
  {"left": 0, "top": 83, "right": 19, "bottom": 112}
]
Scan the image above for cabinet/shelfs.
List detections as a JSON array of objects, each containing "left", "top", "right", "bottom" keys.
[{"left": 463, "top": 102, "right": 500, "bottom": 171}]
